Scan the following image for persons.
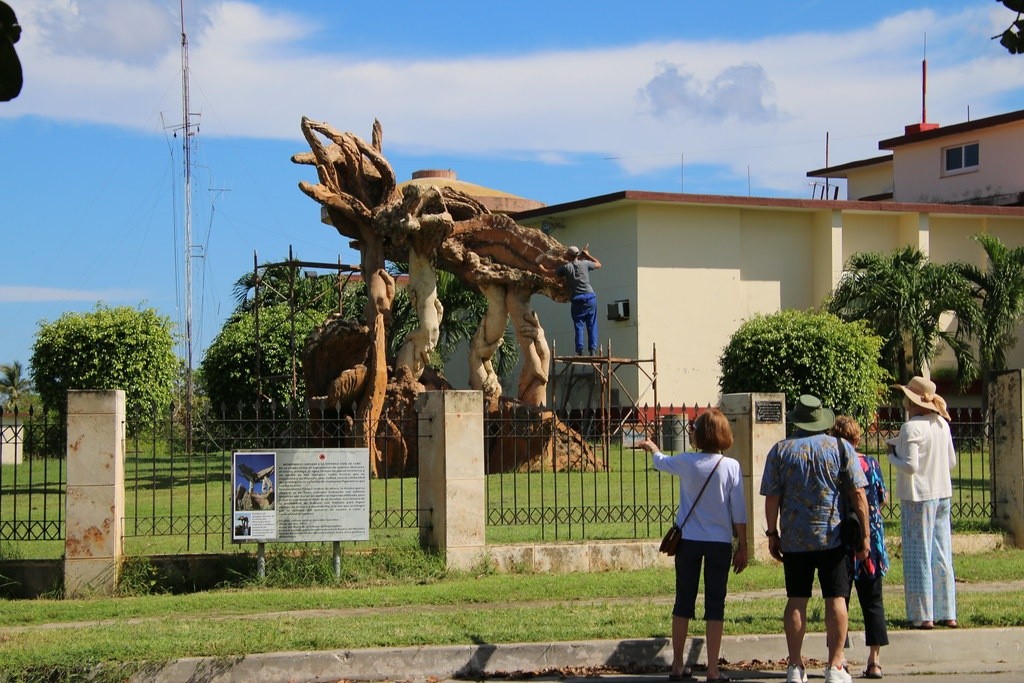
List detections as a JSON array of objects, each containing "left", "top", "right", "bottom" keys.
[
  {"left": 886, "top": 375, "right": 957, "bottom": 631},
  {"left": 538, "top": 245, "right": 601, "bottom": 357},
  {"left": 826, "top": 415, "right": 889, "bottom": 679},
  {"left": 638, "top": 408, "right": 748, "bottom": 683},
  {"left": 758, "top": 394, "right": 871, "bottom": 683}
]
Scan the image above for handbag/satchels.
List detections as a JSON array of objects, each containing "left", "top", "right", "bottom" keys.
[
  {"left": 659, "top": 525, "right": 682, "bottom": 556},
  {"left": 846, "top": 510, "right": 863, "bottom": 544}
]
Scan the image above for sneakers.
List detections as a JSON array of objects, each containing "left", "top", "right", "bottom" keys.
[
  {"left": 824, "top": 665, "right": 852, "bottom": 683},
  {"left": 786, "top": 664, "right": 809, "bottom": 683}
]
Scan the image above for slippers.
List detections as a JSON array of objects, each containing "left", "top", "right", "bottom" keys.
[
  {"left": 669, "top": 666, "right": 693, "bottom": 680},
  {"left": 707, "top": 672, "right": 729, "bottom": 683}
]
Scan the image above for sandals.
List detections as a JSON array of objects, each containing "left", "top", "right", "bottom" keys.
[
  {"left": 938, "top": 619, "right": 959, "bottom": 627},
  {"left": 908, "top": 620, "right": 934, "bottom": 628},
  {"left": 865, "top": 663, "right": 883, "bottom": 678}
]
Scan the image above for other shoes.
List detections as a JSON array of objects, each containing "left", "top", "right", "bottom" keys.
[
  {"left": 576, "top": 349, "right": 583, "bottom": 356},
  {"left": 590, "top": 349, "right": 596, "bottom": 356}
]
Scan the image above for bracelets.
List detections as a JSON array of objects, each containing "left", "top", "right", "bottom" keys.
[{"left": 650, "top": 446, "right": 658, "bottom": 451}]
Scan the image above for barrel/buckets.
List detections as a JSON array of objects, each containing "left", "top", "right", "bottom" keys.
[{"left": 663, "top": 413, "right": 690, "bottom": 452}]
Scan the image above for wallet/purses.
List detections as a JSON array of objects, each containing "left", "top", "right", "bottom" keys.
[{"left": 887, "top": 437, "right": 899, "bottom": 457}]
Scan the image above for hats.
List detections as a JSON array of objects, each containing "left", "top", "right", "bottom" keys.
[
  {"left": 785, "top": 395, "right": 836, "bottom": 432},
  {"left": 567, "top": 246, "right": 579, "bottom": 255},
  {"left": 889, "top": 376, "right": 952, "bottom": 422}
]
